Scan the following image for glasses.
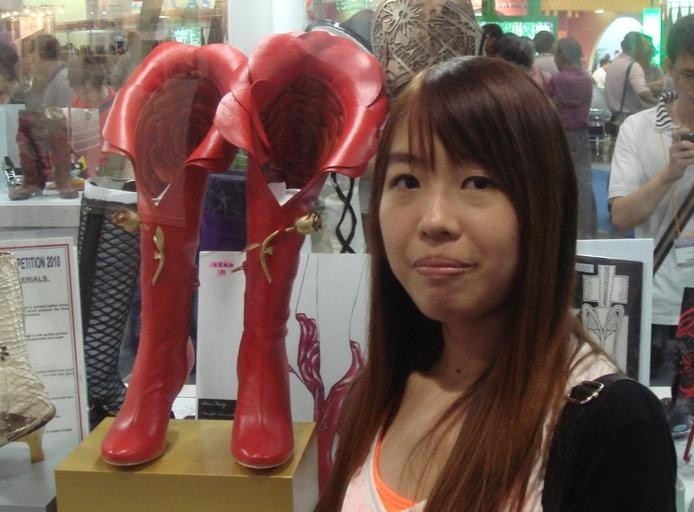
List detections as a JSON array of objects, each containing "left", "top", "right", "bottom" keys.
[{"left": 670, "top": 68, "right": 694, "bottom": 80}]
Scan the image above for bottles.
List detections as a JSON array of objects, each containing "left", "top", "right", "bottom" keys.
[{"left": 587, "top": 109, "right": 606, "bottom": 138}]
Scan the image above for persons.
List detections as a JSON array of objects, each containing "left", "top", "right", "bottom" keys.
[
  {"left": 314, "top": 57, "right": 677, "bottom": 512},
  {"left": 608, "top": 16, "right": 694, "bottom": 387},
  {"left": 482, "top": 24, "right": 598, "bottom": 240},
  {"left": 0, "top": 34, "right": 116, "bottom": 177},
  {"left": 604, "top": 31, "right": 665, "bottom": 141},
  {"left": 592, "top": 50, "right": 620, "bottom": 89}
]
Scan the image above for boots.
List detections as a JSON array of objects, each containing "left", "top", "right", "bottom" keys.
[
  {"left": 77, "top": 192, "right": 140, "bottom": 418},
  {"left": 45, "top": 110, "right": 78, "bottom": 200},
  {"left": 9, "top": 110, "right": 48, "bottom": 200},
  {"left": 0, "top": 251, "right": 57, "bottom": 463}
]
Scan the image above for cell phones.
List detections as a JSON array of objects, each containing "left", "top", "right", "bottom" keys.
[{"left": 681, "top": 133, "right": 694, "bottom": 152}]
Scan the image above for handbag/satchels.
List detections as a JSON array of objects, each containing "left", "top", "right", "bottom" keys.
[{"left": 604, "top": 110, "right": 627, "bottom": 134}]
